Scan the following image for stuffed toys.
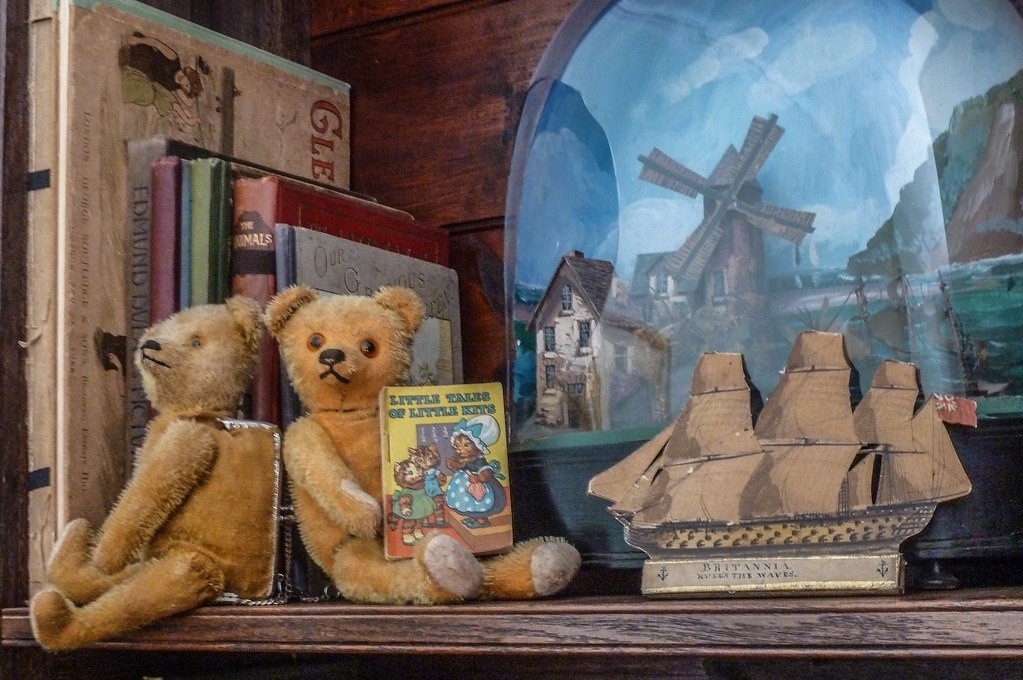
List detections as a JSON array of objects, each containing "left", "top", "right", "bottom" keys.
[
  {"left": 26, "top": 294, "right": 275, "bottom": 654},
  {"left": 263, "top": 283, "right": 583, "bottom": 606}
]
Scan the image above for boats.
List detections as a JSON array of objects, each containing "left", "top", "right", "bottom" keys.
[{"left": 586, "top": 329, "right": 977, "bottom": 596}]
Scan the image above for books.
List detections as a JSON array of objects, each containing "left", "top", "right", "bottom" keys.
[
  {"left": 378, "top": 382, "right": 514, "bottom": 561},
  {"left": 25, "top": 0, "right": 466, "bottom": 605}
]
